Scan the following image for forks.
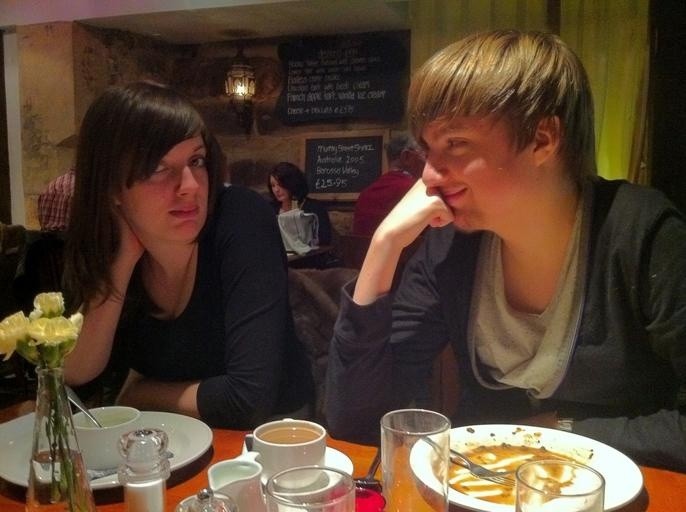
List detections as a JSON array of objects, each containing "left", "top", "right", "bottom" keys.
[
  {"left": 395, "top": 420, "right": 512, "bottom": 481},
  {"left": 350, "top": 441, "right": 382, "bottom": 490}
]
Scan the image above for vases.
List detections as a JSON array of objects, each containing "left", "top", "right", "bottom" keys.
[{"left": 24, "top": 366, "right": 96, "bottom": 511}]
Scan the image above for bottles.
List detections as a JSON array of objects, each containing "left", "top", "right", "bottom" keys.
[{"left": 114, "top": 428, "right": 173, "bottom": 512}]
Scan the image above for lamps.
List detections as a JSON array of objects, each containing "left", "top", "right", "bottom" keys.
[{"left": 223, "top": 30, "right": 258, "bottom": 127}]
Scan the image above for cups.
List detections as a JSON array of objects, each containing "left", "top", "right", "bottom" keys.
[
  {"left": 263, "top": 467, "right": 372, "bottom": 505},
  {"left": 251, "top": 417, "right": 325, "bottom": 487},
  {"left": 514, "top": 457, "right": 606, "bottom": 512},
  {"left": 378, "top": 407, "right": 451, "bottom": 512},
  {"left": 65, "top": 405, "right": 144, "bottom": 471}
]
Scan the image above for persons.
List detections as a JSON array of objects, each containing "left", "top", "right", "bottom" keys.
[
  {"left": 347, "top": 128, "right": 431, "bottom": 265},
  {"left": 259, "top": 158, "right": 338, "bottom": 269},
  {"left": 326, "top": 27, "right": 686, "bottom": 479},
  {"left": 42, "top": 78, "right": 313, "bottom": 430},
  {"left": 34, "top": 170, "right": 74, "bottom": 234}
]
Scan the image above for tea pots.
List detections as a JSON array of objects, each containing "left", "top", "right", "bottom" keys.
[{"left": 206, "top": 456, "right": 265, "bottom": 512}]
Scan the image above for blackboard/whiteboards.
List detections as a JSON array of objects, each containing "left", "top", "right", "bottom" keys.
[
  {"left": 305, "top": 136, "right": 383, "bottom": 192},
  {"left": 281, "top": 30, "right": 411, "bottom": 123}
]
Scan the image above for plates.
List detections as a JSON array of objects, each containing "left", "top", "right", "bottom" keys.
[
  {"left": 407, "top": 423, "right": 645, "bottom": 511},
  {"left": 0, "top": 405, "right": 213, "bottom": 491},
  {"left": 234, "top": 445, "right": 356, "bottom": 498}
]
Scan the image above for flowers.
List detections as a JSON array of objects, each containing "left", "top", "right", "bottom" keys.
[{"left": 0, "top": 290, "right": 84, "bottom": 511}]
